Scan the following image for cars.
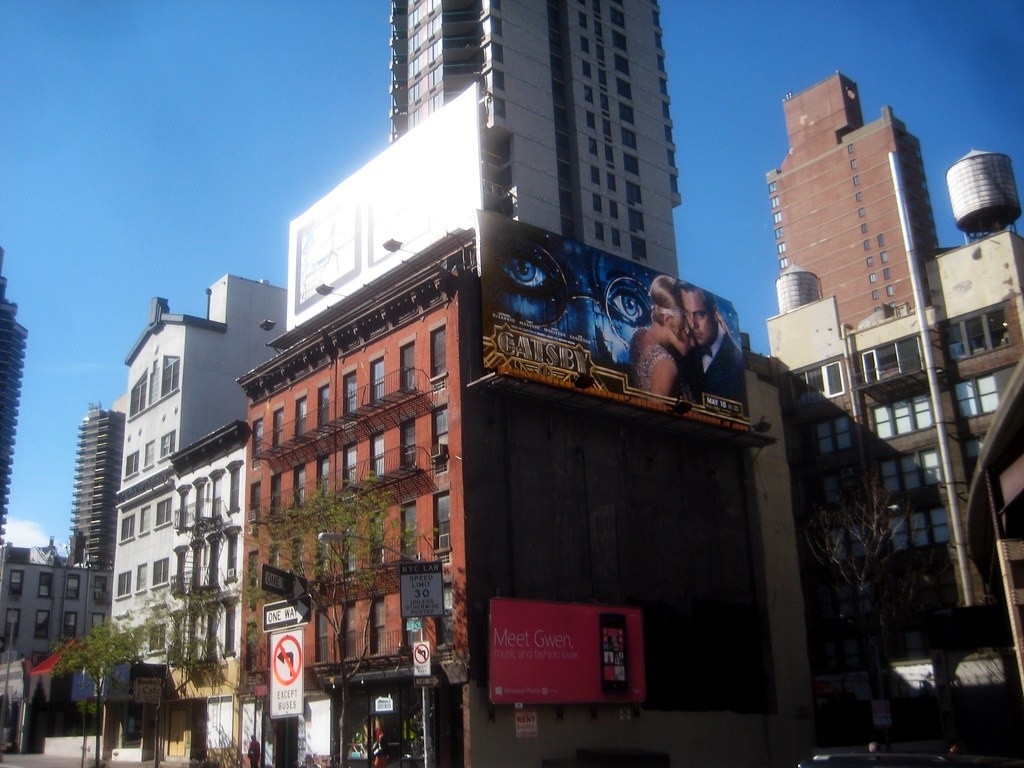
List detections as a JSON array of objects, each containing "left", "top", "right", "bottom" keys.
[{"left": 797, "top": 747, "right": 1024, "bottom": 768}]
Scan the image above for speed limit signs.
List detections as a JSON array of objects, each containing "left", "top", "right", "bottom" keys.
[{"left": 401, "top": 562, "right": 444, "bottom": 618}]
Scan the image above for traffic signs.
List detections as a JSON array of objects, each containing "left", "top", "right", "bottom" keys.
[
  {"left": 262, "top": 564, "right": 308, "bottom": 601},
  {"left": 411, "top": 671, "right": 441, "bottom": 689},
  {"left": 262, "top": 600, "right": 310, "bottom": 634}
]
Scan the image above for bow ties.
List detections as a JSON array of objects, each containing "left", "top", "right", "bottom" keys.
[{"left": 701, "top": 345, "right": 712, "bottom": 358}]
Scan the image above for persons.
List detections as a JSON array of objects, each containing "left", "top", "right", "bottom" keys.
[
  {"left": 247, "top": 735, "right": 260, "bottom": 768},
  {"left": 869, "top": 742, "right": 884, "bottom": 753},
  {"left": 373, "top": 734, "right": 391, "bottom": 768},
  {"left": 627, "top": 275, "right": 749, "bottom": 417}
]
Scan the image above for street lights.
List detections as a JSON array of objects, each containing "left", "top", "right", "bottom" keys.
[
  {"left": 318, "top": 531, "right": 430, "bottom": 768},
  {"left": 870, "top": 502, "right": 903, "bottom": 750}
]
